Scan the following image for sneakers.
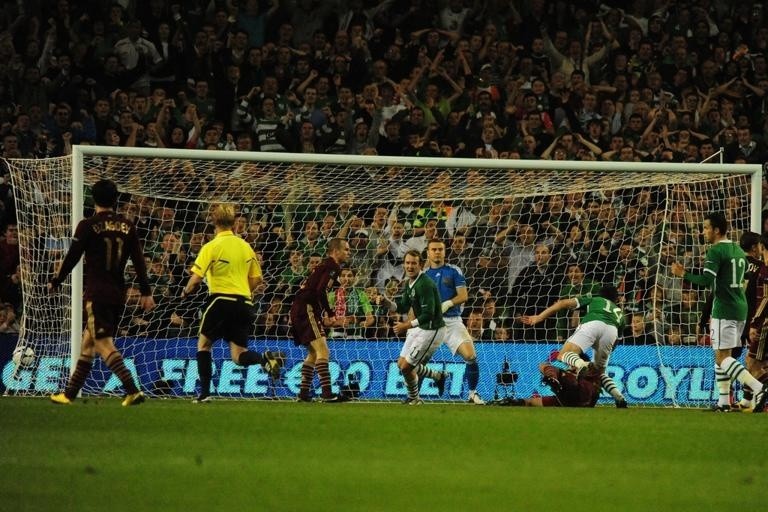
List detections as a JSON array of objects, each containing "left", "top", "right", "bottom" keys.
[
  {"left": 121, "top": 391, "right": 146, "bottom": 407},
  {"left": 319, "top": 395, "right": 353, "bottom": 403},
  {"left": 468, "top": 391, "right": 486, "bottom": 404},
  {"left": 262, "top": 350, "right": 280, "bottom": 380},
  {"left": 576, "top": 362, "right": 594, "bottom": 380},
  {"left": 615, "top": 397, "right": 628, "bottom": 407},
  {"left": 733, "top": 402, "right": 753, "bottom": 412},
  {"left": 192, "top": 395, "right": 213, "bottom": 404},
  {"left": 434, "top": 371, "right": 450, "bottom": 397},
  {"left": 50, "top": 393, "right": 70, "bottom": 404},
  {"left": 753, "top": 385, "right": 767, "bottom": 414},
  {"left": 402, "top": 398, "right": 424, "bottom": 405},
  {"left": 544, "top": 376, "right": 563, "bottom": 393},
  {"left": 296, "top": 394, "right": 317, "bottom": 402},
  {"left": 703, "top": 405, "right": 735, "bottom": 414}
]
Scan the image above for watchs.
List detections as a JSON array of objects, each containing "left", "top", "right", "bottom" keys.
[{"left": 181, "top": 287, "right": 188, "bottom": 297}]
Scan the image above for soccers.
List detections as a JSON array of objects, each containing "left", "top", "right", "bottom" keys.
[{"left": 12, "top": 345, "right": 35, "bottom": 369}]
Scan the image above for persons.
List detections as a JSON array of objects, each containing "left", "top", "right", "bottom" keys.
[
  {"left": 348, "top": 229, "right": 374, "bottom": 286},
  {"left": 252, "top": 250, "right": 272, "bottom": 275},
  {"left": 276, "top": 247, "right": 307, "bottom": 295},
  {"left": 261, "top": 297, "right": 288, "bottom": 325},
  {"left": 326, "top": 212, "right": 453, "bottom": 235},
  {"left": 271, "top": 226, "right": 285, "bottom": 240},
  {"left": 232, "top": 213, "right": 248, "bottom": 238},
  {"left": 729, "top": 231, "right": 768, "bottom": 412},
  {"left": 462, "top": 309, "right": 491, "bottom": 342},
  {"left": 146, "top": 231, "right": 193, "bottom": 269},
  {"left": 554, "top": 263, "right": 615, "bottom": 344},
  {"left": 126, "top": 253, "right": 172, "bottom": 307},
  {"left": 0, "top": 0, "right": 768, "bottom": 212},
  {"left": 293, "top": 252, "right": 322, "bottom": 293},
  {"left": 125, "top": 192, "right": 159, "bottom": 237},
  {"left": 560, "top": 221, "right": 590, "bottom": 267},
  {"left": 402, "top": 239, "right": 486, "bottom": 406},
  {"left": 611, "top": 212, "right": 705, "bottom": 288},
  {"left": 520, "top": 284, "right": 628, "bottom": 408},
  {"left": 475, "top": 298, "right": 505, "bottom": 328},
  {"left": 151, "top": 208, "right": 189, "bottom": 252},
  {"left": 113, "top": 283, "right": 152, "bottom": 338},
  {"left": 251, "top": 279, "right": 270, "bottom": 312},
  {"left": 0, "top": 213, "right": 69, "bottom": 332},
  {"left": 671, "top": 212, "right": 768, "bottom": 413},
  {"left": 180, "top": 205, "right": 286, "bottom": 404},
  {"left": 323, "top": 266, "right": 375, "bottom": 341},
  {"left": 730, "top": 232, "right": 763, "bottom": 409},
  {"left": 464, "top": 206, "right": 518, "bottom": 253},
  {"left": 375, "top": 249, "right": 452, "bottom": 407},
  {"left": 46, "top": 178, "right": 156, "bottom": 407},
  {"left": 508, "top": 240, "right": 567, "bottom": 343},
  {"left": 445, "top": 233, "right": 477, "bottom": 283},
  {"left": 492, "top": 323, "right": 511, "bottom": 341},
  {"left": 250, "top": 311, "right": 284, "bottom": 340},
  {"left": 491, "top": 349, "right": 601, "bottom": 407},
  {"left": 495, "top": 219, "right": 564, "bottom": 294},
  {"left": 292, "top": 219, "right": 328, "bottom": 254},
  {"left": 288, "top": 237, "right": 351, "bottom": 404},
  {"left": 170, "top": 259, "right": 209, "bottom": 327},
  {"left": 620, "top": 288, "right": 708, "bottom": 343},
  {"left": 178, "top": 231, "right": 209, "bottom": 268},
  {"left": 519, "top": 198, "right": 549, "bottom": 246}
]
